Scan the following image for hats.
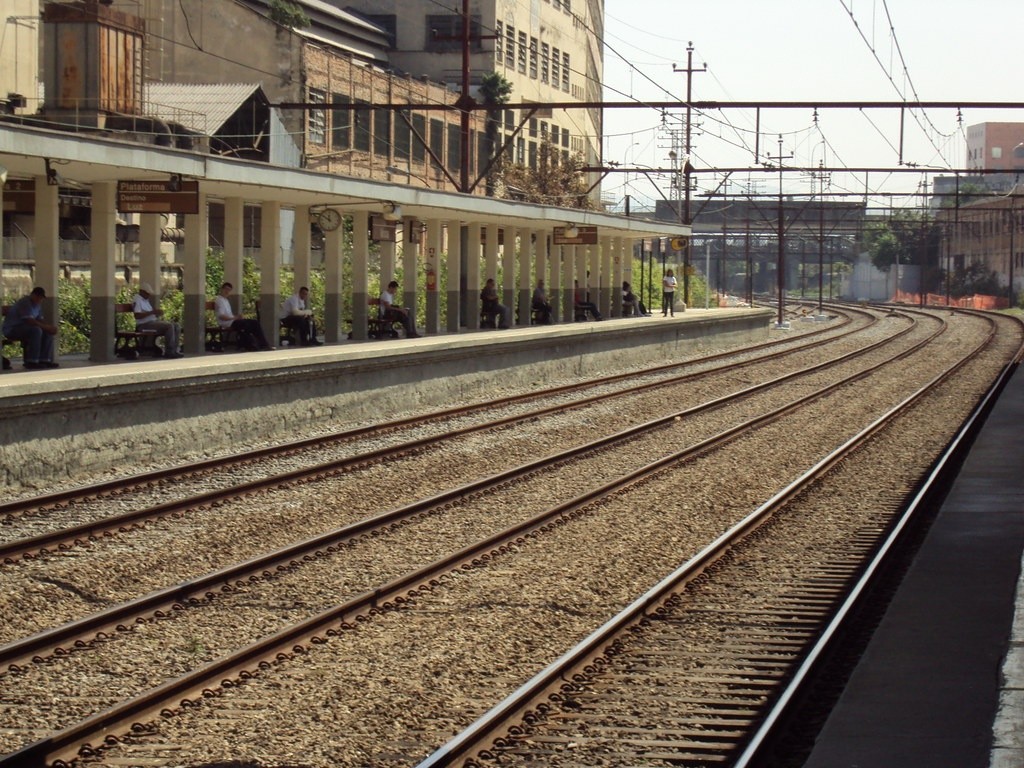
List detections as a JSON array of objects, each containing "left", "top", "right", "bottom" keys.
[
  {"left": 140, "top": 283, "right": 154, "bottom": 293},
  {"left": 32, "top": 287, "right": 47, "bottom": 298}
]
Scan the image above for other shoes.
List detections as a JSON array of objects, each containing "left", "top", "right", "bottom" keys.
[
  {"left": 407, "top": 332, "right": 422, "bottom": 338},
  {"left": 498, "top": 323, "right": 510, "bottom": 329},
  {"left": 26, "top": 361, "right": 57, "bottom": 369},
  {"left": 301, "top": 337, "right": 312, "bottom": 346},
  {"left": 264, "top": 344, "right": 277, "bottom": 350},
  {"left": 310, "top": 338, "right": 323, "bottom": 345}
]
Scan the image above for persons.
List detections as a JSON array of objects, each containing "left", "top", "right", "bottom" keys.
[
  {"left": 279, "top": 287, "right": 324, "bottom": 347},
  {"left": 639, "top": 300, "right": 651, "bottom": 316},
  {"left": 533, "top": 279, "right": 555, "bottom": 324},
  {"left": 3, "top": 287, "right": 61, "bottom": 370},
  {"left": 380, "top": 280, "right": 423, "bottom": 338},
  {"left": 623, "top": 281, "right": 645, "bottom": 317},
  {"left": 130, "top": 282, "right": 184, "bottom": 358},
  {"left": 575, "top": 280, "right": 603, "bottom": 321},
  {"left": 480, "top": 278, "right": 510, "bottom": 329},
  {"left": 214, "top": 282, "right": 276, "bottom": 351},
  {"left": 662, "top": 269, "right": 677, "bottom": 318}
]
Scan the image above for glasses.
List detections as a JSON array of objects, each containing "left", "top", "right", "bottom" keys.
[{"left": 539, "top": 283, "right": 545, "bottom": 285}]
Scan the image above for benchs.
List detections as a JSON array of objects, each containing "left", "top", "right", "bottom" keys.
[
  {"left": 114, "top": 302, "right": 166, "bottom": 360},
  {"left": 572, "top": 302, "right": 593, "bottom": 322},
  {"left": 608, "top": 302, "right": 636, "bottom": 318},
  {"left": 514, "top": 307, "right": 546, "bottom": 324},
  {"left": 278, "top": 319, "right": 322, "bottom": 346},
  {"left": 343, "top": 318, "right": 399, "bottom": 341},
  {"left": 0, "top": 306, "right": 26, "bottom": 349},
  {"left": 205, "top": 300, "right": 254, "bottom": 352},
  {"left": 479, "top": 310, "right": 498, "bottom": 329}
]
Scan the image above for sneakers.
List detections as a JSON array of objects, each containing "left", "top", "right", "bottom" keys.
[{"left": 165, "top": 348, "right": 184, "bottom": 358}]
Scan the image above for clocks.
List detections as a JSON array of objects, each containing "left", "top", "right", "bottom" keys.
[{"left": 318, "top": 208, "right": 343, "bottom": 231}]
